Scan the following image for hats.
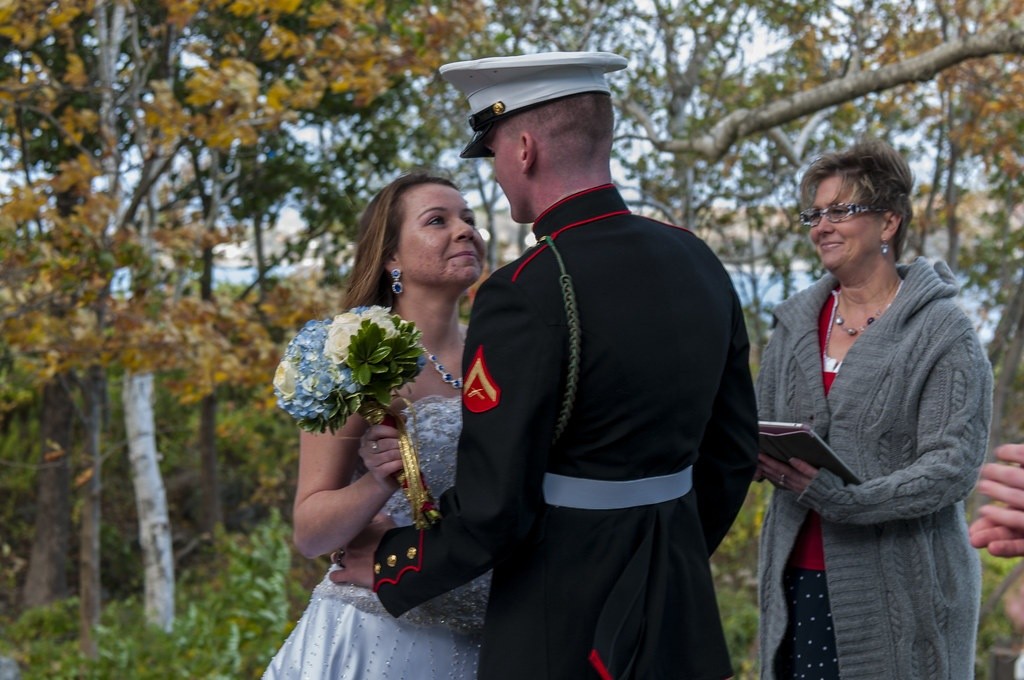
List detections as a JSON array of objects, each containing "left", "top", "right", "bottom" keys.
[{"left": 439, "top": 51, "right": 630, "bottom": 159}]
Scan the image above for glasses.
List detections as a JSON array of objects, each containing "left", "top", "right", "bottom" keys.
[{"left": 800, "top": 204, "right": 889, "bottom": 226}]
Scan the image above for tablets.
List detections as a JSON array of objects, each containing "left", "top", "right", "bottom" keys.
[{"left": 756, "top": 420, "right": 862, "bottom": 489}]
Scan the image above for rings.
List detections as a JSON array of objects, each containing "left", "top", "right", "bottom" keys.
[
  {"left": 335, "top": 549, "right": 345, "bottom": 568},
  {"left": 371, "top": 441, "right": 379, "bottom": 454},
  {"left": 778, "top": 474, "right": 785, "bottom": 488}
]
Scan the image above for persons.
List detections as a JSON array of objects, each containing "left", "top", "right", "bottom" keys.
[
  {"left": 969, "top": 443, "right": 1024, "bottom": 559},
  {"left": 260, "top": 175, "right": 494, "bottom": 680},
  {"left": 754, "top": 133, "right": 993, "bottom": 680},
  {"left": 330, "top": 53, "right": 760, "bottom": 680}
]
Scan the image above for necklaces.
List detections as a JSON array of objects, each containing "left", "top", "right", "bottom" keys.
[
  {"left": 419, "top": 344, "right": 463, "bottom": 388},
  {"left": 835, "top": 277, "right": 900, "bottom": 335}
]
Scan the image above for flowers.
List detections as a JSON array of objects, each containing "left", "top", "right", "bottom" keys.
[{"left": 271, "top": 305, "right": 443, "bottom": 527}]
